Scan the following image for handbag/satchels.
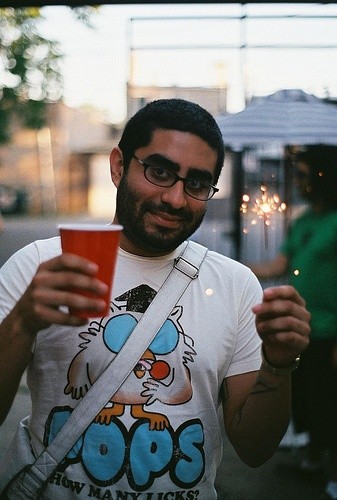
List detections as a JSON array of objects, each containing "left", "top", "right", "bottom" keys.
[{"left": 0, "top": 415, "right": 44, "bottom": 500}]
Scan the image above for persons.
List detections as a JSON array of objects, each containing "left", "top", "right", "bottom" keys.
[
  {"left": 0, "top": 99, "right": 312, "bottom": 500},
  {"left": 246, "top": 146, "right": 337, "bottom": 479}
]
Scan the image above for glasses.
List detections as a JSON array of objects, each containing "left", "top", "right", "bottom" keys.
[
  {"left": 128, "top": 146, "right": 219, "bottom": 202},
  {"left": 293, "top": 169, "right": 315, "bottom": 180}
]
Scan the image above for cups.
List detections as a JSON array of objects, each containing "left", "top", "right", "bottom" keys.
[{"left": 57, "top": 224, "right": 124, "bottom": 318}]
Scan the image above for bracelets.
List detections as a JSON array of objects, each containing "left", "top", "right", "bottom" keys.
[{"left": 261, "top": 341, "right": 301, "bottom": 376}]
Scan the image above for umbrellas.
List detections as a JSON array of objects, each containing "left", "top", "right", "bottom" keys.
[{"left": 216, "top": 86, "right": 334, "bottom": 262}]
